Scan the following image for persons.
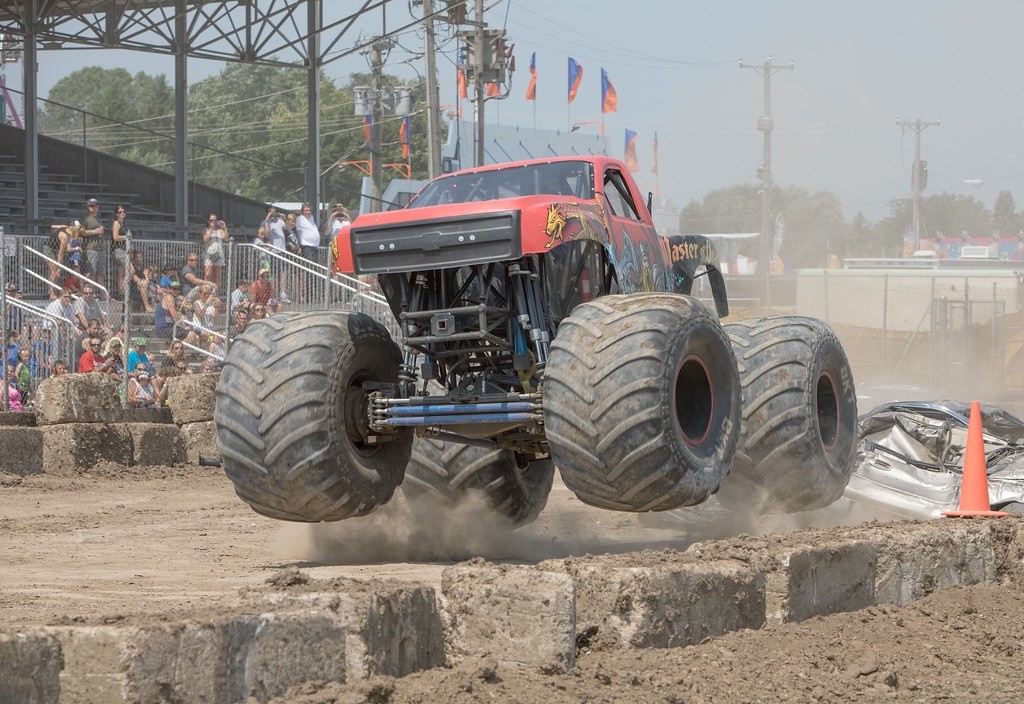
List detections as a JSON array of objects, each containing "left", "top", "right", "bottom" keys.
[{"left": 0, "top": 199, "right": 353, "bottom": 412}]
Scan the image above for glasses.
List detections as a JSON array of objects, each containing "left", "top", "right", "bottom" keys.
[
  {"left": 173, "top": 346, "right": 183, "bottom": 350},
  {"left": 254, "top": 308, "right": 264, "bottom": 311},
  {"left": 335, "top": 205, "right": 343, "bottom": 207},
  {"left": 117, "top": 211, "right": 125, "bottom": 214},
  {"left": 112, "top": 344, "right": 121, "bottom": 348},
  {"left": 172, "top": 288, "right": 180, "bottom": 291},
  {"left": 63, "top": 296, "right": 71, "bottom": 298},
  {"left": 136, "top": 367, "right": 147, "bottom": 372},
  {"left": 83, "top": 292, "right": 93, "bottom": 295},
  {"left": 209, "top": 219, "right": 217, "bottom": 222},
  {"left": 91, "top": 344, "right": 101, "bottom": 346}
]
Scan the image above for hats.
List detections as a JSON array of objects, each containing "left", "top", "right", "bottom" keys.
[
  {"left": 109, "top": 338, "right": 121, "bottom": 346},
  {"left": 138, "top": 372, "right": 149, "bottom": 379},
  {"left": 86, "top": 198, "right": 99, "bottom": 207},
  {"left": 266, "top": 298, "right": 279, "bottom": 307},
  {"left": 169, "top": 281, "right": 181, "bottom": 288},
  {"left": 70, "top": 221, "right": 81, "bottom": 227},
  {"left": 333, "top": 203, "right": 347, "bottom": 211},
  {"left": 133, "top": 338, "right": 147, "bottom": 345},
  {"left": 258, "top": 269, "right": 270, "bottom": 276}
]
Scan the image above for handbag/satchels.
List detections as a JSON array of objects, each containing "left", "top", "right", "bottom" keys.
[{"left": 210, "top": 252, "right": 221, "bottom": 262}]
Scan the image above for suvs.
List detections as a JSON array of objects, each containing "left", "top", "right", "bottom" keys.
[{"left": 213, "top": 152, "right": 862, "bottom": 523}]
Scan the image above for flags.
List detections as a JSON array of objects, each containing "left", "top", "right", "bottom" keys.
[
  {"left": 601, "top": 69, "right": 617, "bottom": 112},
  {"left": 457, "top": 49, "right": 467, "bottom": 99},
  {"left": 400, "top": 116, "right": 411, "bottom": 158},
  {"left": 624, "top": 129, "right": 638, "bottom": 172},
  {"left": 526, "top": 52, "right": 536, "bottom": 100},
  {"left": 568, "top": 57, "right": 583, "bottom": 102},
  {"left": 363, "top": 115, "right": 372, "bottom": 143}
]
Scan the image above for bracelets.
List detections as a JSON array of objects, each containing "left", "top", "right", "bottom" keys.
[
  {"left": 103, "top": 363, "right": 107, "bottom": 367},
  {"left": 93, "top": 230, "right": 95, "bottom": 235},
  {"left": 164, "top": 384, "right": 168, "bottom": 387}
]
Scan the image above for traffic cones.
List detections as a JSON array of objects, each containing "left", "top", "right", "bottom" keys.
[{"left": 941, "top": 401, "right": 1012, "bottom": 517}]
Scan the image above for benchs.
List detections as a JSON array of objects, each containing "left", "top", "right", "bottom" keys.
[{"left": 0, "top": 151, "right": 259, "bottom": 366}]
